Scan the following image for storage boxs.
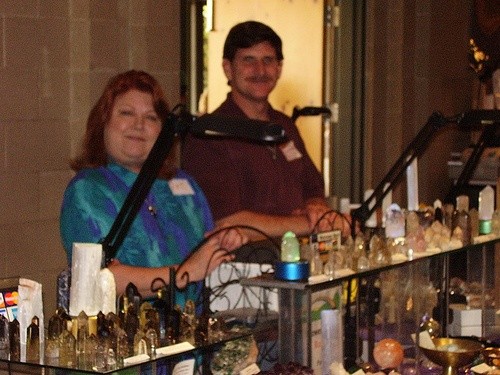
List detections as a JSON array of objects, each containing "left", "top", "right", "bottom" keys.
[{"left": 278, "top": 285, "right": 343, "bottom": 375}]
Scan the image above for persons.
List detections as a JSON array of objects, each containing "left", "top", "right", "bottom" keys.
[
  {"left": 59, "top": 69, "right": 249, "bottom": 375},
  {"left": 180, "top": 21, "right": 352, "bottom": 371}
]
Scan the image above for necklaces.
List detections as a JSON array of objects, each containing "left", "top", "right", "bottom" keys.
[{"left": 106, "top": 168, "right": 158, "bottom": 218}]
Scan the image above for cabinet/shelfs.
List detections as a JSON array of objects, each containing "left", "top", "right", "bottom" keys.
[{"left": 0, "top": 235, "right": 500, "bottom": 375}]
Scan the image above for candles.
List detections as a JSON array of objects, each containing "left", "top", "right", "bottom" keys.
[
  {"left": 479, "top": 184, "right": 494, "bottom": 221},
  {"left": 364, "top": 189, "right": 377, "bottom": 228},
  {"left": 406, "top": 155, "right": 419, "bottom": 213},
  {"left": 381, "top": 182, "right": 392, "bottom": 228},
  {"left": 340, "top": 198, "right": 351, "bottom": 214},
  {"left": 455, "top": 194, "right": 469, "bottom": 212}
]
